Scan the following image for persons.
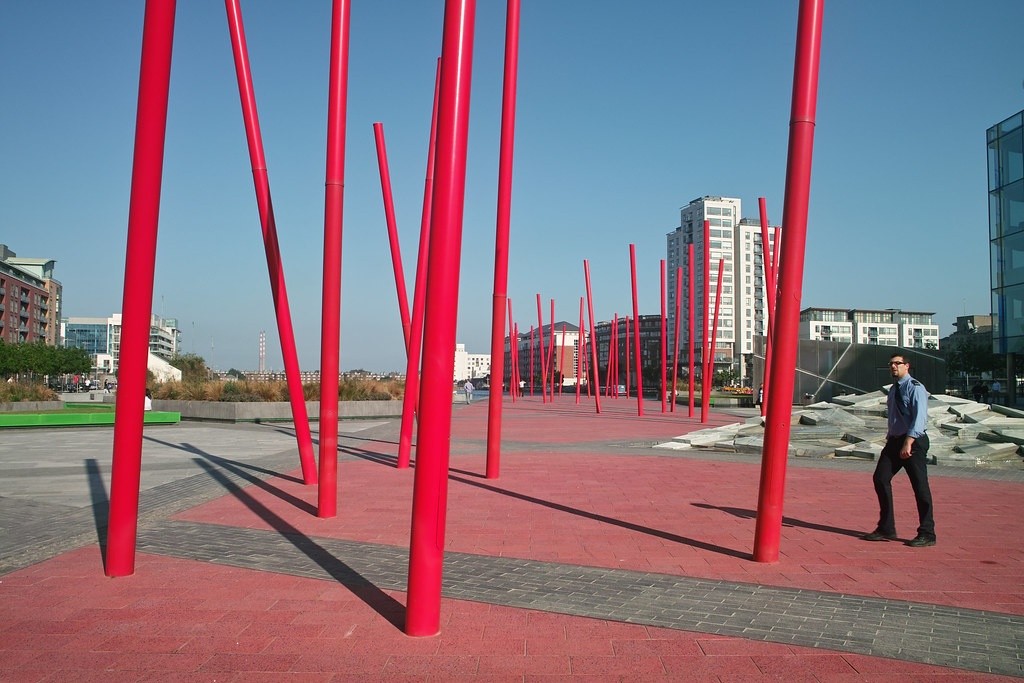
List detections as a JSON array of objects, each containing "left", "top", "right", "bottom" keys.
[
  {"left": 519, "top": 379, "right": 526, "bottom": 397},
  {"left": 865, "top": 354, "right": 936, "bottom": 547},
  {"left": 7, "top": 376, "right": 14, "bottom": 384},
  {"left": 144, "top": 388, "right": 153, "bottom": 411},
  {"left": 73, "top": 374, "right": 79, "bottom": 393},
  {"left": 464, "top": 379, "right": 474, "bottom": 404},
  {"left": 104, "top": 379, "right": 111, "bottom": 393},
  {"left": 758, "top": 383, "right": 764, "bottom": 410},
  {"left": 721, "top": 385, "right": 754, "bottom": 394},
  {"left": 84, "top": 378, "right": 92, "bottom": 392},
  {"left": 668, "top": 389, "right": 679, "bottom": 402},
  {"left": 971, "top": 380, "right": 990, "bottom": 402},
  {"left": 992, "top": 378, "right": 1001, "bottom": 403}
]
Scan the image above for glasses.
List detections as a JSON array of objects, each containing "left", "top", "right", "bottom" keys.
[{"left": 887, "top": 361, "right": 906, "bottom": 366}]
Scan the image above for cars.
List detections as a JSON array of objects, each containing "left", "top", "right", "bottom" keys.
[{"left": 42, "top": 380, "right": 103, "bottom": 392}]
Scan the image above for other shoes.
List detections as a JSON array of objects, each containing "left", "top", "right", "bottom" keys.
[{"left": 467, "top": 402, "right": 469, "bottom": 404}]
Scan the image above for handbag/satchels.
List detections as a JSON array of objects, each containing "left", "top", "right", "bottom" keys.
[{"left": 756, "top": 398, "right": 759, "bottom": 404}]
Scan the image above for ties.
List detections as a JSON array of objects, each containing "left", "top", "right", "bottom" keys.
[{"left": 894, "top": 381, "right": 911, "bottom": 417}]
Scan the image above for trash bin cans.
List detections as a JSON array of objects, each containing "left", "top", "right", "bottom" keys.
[{"left": 802, "top": 393, "right": 816, "bottom": 406}]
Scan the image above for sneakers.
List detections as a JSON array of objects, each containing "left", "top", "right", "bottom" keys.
[
  {"left": 906, "top": 534, "right": 937, "bottom": 548},
  {"left": 863, "top": 527, "right": 897, "bottom": 540}
]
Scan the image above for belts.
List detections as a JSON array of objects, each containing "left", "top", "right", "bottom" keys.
[{"left": 889, "top": 435, "right": 905, "bottom": 439}]
